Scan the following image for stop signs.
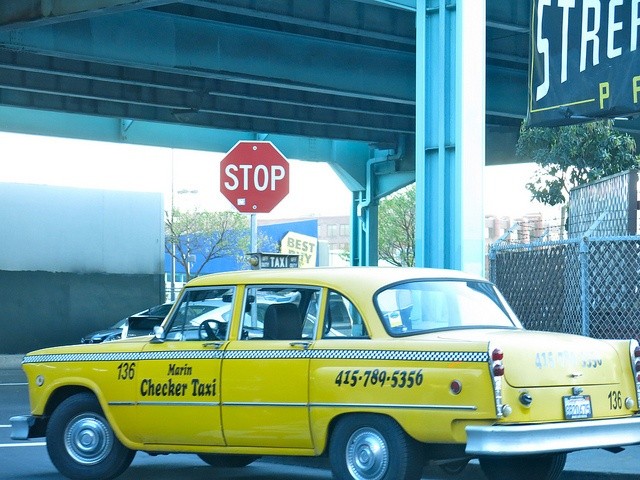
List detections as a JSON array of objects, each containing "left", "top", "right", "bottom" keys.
[{"left": 219, "top": 141, "right": 290, "bottom": 212}]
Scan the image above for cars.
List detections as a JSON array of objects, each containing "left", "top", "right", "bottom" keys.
[
  {"left": 12, "top": 256, "right": 640, "bottom": 480},
  {"left": 78, "top": 296, "right": 344, "bottom": 344}
]
